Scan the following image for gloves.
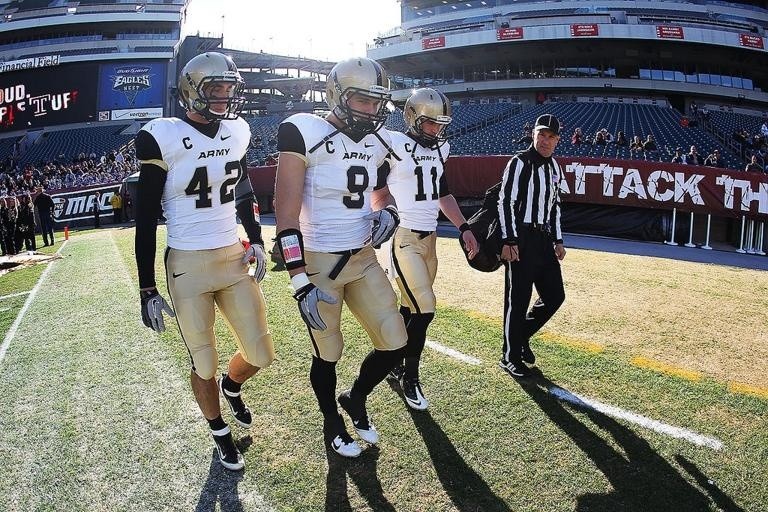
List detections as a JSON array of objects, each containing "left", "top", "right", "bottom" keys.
[
  {"left": 363, "top": 207, "right": 400, "bottom": 247},
  {"left": 293, "top": 283, "right": 336, "bottom": 330},
  {"left": 242, "top": 242, "right": 266, "bottom": 283},
  {"left": 140, "top": 288, "right": 175, "bottom": 332}
]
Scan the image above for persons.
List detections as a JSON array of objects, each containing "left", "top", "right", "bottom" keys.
[
  {"left": 93, "top": 192, "right": 101, "bottom": 229},
  {"left": 132, "top": 51, "right": 278, "bottom": 472},
  {"left": 672, "top": 145, "right": 720, "bottom": 166},
  {"left": 111, "top": 191, "right": 133, "bottom": 223},
  {"left": 385, "top": 87, "right": 480, "bottom": 414},
  {"left": 499, "top": 115, "right": 567, "bottom": 381},
  {"left": 732, "top": 122, "right": 767, "bottom": 149},
  {"left": 572, "top": 127, "right": 658, "bottom": 151},
  {"left": 746, "top": 155, "right": 763, "bottom": 173},
  {"left": 0, "top": 152, "right": 138, "bottom": 196},
  {"left": 274, "top": 58, "right": 409, "bottom": 460},
  {"left": 691, "top": 103, "right": 708, "bottom": 118},
  {"left": 248, "top": 135, "right": 277, "bottom": 167},
  {"left": 0, "top": 188, "right": 54, "bottom": 254},
  {"left": 681, "top": 115, "right": 688, "bottom": 127},
  {"left": 518, "top": 122, "right": 533, "bottom": 144}
]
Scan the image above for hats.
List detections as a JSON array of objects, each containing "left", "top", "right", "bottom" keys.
[{"left": 535, "top": 115, "right": 560, "bottom": 135}]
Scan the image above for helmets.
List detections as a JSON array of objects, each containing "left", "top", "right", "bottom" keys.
[
  {"left": 177, "top": 52, "right": 246, "bottom": 121},
  {"left": 403, "top": 87, "right": 452, "bottom": 145},
  {"left": 324, "top": 57, "right": 391, "bottom": 133}
]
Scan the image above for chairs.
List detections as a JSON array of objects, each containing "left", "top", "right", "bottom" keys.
[
  {"left": 381, "top": 98, "right": 768, "bottom": 174},
  {"left": 0, "top": 104, "right": 289, "bottom": 191}
]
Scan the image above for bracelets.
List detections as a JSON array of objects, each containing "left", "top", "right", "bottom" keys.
[
  {"left": 457, "top": 222, "right": 474, "bottom": 236},
  {"left": 289, "top": 270, "right": 309, "bottom": 289}
]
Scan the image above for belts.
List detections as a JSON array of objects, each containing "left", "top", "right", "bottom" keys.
[
  {"left": 411, "top": 229, "right": 433, "bottom": 239},
  {"left": 328, "top": 248, "right": 362, "bottom": 280}
]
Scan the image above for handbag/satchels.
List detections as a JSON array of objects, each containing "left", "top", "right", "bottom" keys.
[{"left": 459, "top": 181, "right": 502, "bottom": 272}]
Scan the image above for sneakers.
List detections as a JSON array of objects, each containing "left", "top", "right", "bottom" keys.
[
  {"left": 499, "top": 357, "right": 524, "bottom": 376},
  {"left": 338, "top": 390, "right": 379, "bottom": 444},
  {"left": 521, "top": 342, "right": 535, "bottom": 364},
  {"left": 387, "top": 366, "right": 429, "bottom": 410},
  {"left": 216, "top": 374, "right": 253, "bottom": 428},
  {"left": 324, "top": 415, "right": 361, "bottom": 458},
  {"left": 210, "top": 422, "right": 245, "bottom": 470}
]
